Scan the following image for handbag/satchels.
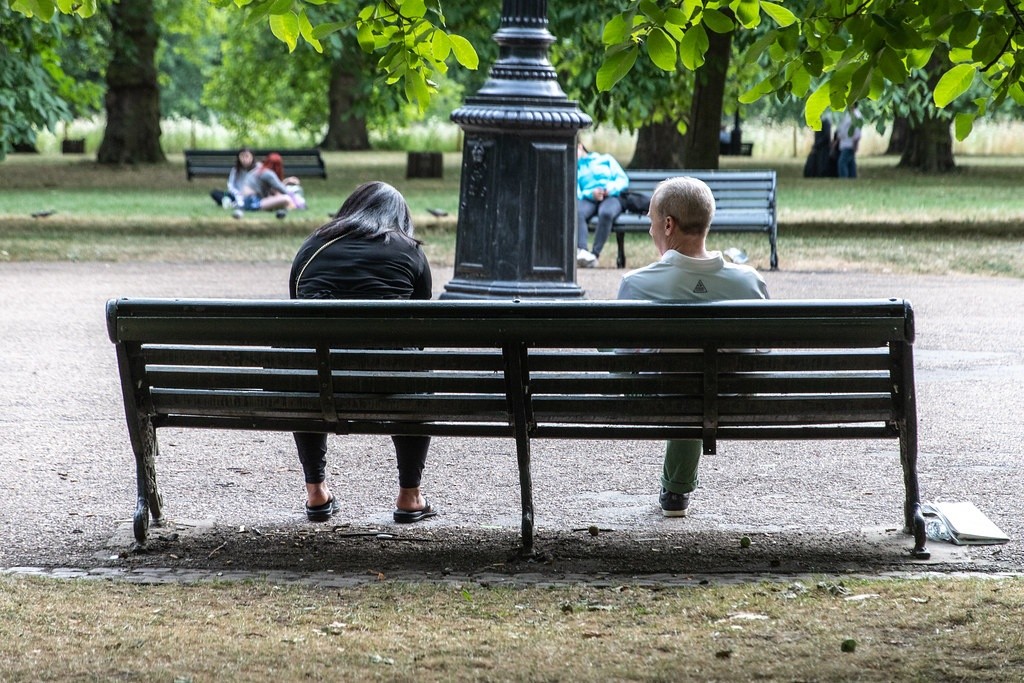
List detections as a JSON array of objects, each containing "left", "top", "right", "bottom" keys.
[{"left": 619, "top": 190, "right": 651, "bottom": 213}]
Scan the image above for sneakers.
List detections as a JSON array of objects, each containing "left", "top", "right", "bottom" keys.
[{"left": 659, "top": 487, "right": 691, "bottom": 516}]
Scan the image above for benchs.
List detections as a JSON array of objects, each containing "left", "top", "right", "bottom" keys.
[
  {"left": 184, "top": 149, "right": 327, "bottom": 181},
  {"left": 719, "top": 141, "right": 753, "bottom": 155},
  {"left": 587, "top": 167, "right": 779, "bottom": 269},
  {"left": 104, "top": 298, "right": 931, "bottom": 560}
]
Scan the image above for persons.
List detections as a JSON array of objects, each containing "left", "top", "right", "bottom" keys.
[
  {"left": 240, "top": 152, "right": 301, "bottom": 210},
  {"left": 830, "top": 98, "right": 863, "bottom": 178},
  {"left": 289, "top": 179, "right": 439, "bottom": 525},
  {"left": 720, "top": 125, "right": 731, "bottom": 143},
  {"left": 209, "top": 147, "right": 256, "bottom": 210},
  {"left": 617, "top": 173, "right": 770, "bottom": 519},
  {"left": 576, "top": 142, "right": 629, "bottom": 269}
]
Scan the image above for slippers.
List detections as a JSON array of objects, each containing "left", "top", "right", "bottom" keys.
[
  {"left": 305, "top": 493, "right": 340, "bottom": 521},
  {"left": 393, "top": 498, "right": 437, "bottom": 521}
]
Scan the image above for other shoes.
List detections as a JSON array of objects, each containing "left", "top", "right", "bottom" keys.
[{"left": 577, "top": 249, "right": 598, "bottom": 269}]
